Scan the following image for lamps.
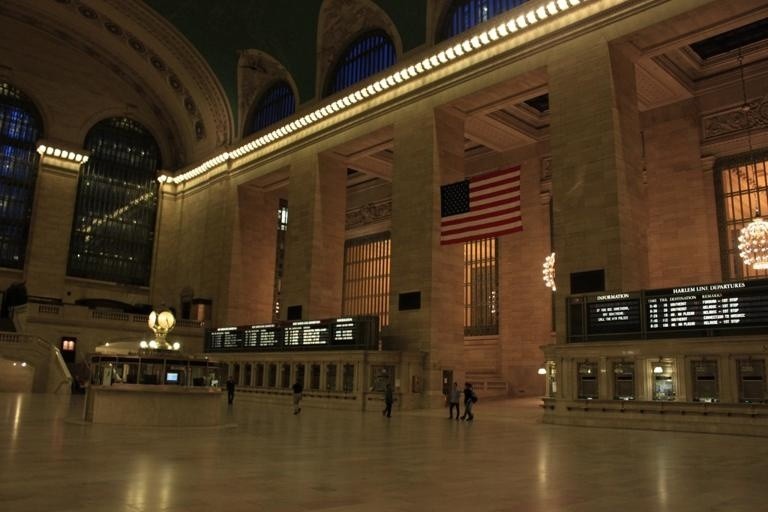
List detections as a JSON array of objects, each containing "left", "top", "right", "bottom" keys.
[
  {"left": 653, "top": 359, "right": 664, "bottom": 373},
  {"left": 542, "top": 251, "right": 557, "bottom": 292},
  {"left": 736, "top": 55, "right": 768, "bottom": 270},
  {"left": 537, "top": 367, "right": 548, "bottom": 375}
]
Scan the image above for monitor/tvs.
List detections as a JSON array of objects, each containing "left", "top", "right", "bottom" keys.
[
  {"left": 167, "top": 373, "right": 178, "bottom": 381},
  {"left": 399, "top": 291, "right": 421, "bottom": 311},
  {"left": 571, "top": 269, "right": 605, "bottom": 294},
  {"left": 287, "top": 305, "right": 302, "bottom": 320}
]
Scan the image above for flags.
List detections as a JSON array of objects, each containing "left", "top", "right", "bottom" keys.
[{"left": 439, "top": 163, "right": 522, "bottom": 246}]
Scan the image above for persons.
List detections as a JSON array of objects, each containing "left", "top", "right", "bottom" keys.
[
  {"left": 383, "top": 384, "right": 393, "bottom": 418},
  {"left": 460, "top": 382, "right": 478, "bottom": 422},
  {"left": 291, "top": 381, "right": 303, "bottom": 416},
  {"left": 225, "top": 376, "right": 235, "bottom": 404},
  {"left": 447, "top": 381, "right": 460, "bottom": 420}
]
